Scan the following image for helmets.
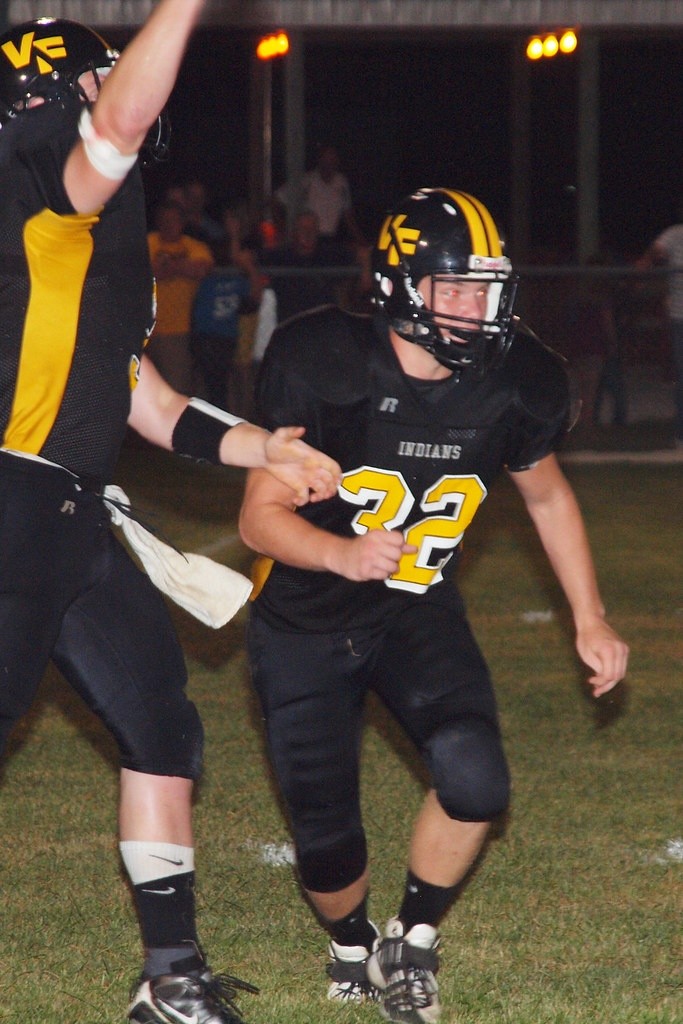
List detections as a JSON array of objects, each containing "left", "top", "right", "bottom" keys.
[
  {"left": 370, "top": 183, "right": 521, "bottom": 361},
  {"left": 1, "top": 19, "right": 165, "bottom": 157}
]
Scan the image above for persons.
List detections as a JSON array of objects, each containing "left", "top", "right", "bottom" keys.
[
  {"left": 236, "top": 186, "right": 627, "bottom": 1024},
  {"left": 148, "top": 130, "right": 679, "bottom": 483},
  {"left": 0, "top": 1, "right": 264, "bottom": 1023}
]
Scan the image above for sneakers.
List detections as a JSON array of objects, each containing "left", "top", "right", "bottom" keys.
[
  {"left": 326, "top": 923, "right": 380, "bottom": 1015},
  {"left": 369, "top": 914, "right": 444, "bottom": 1024},
  {"left": 126, "top": 959, "right": 248, "bottom": 1023}
]
